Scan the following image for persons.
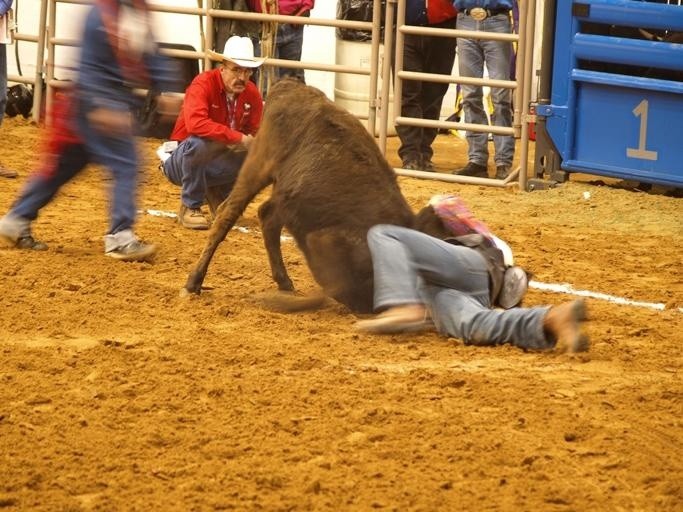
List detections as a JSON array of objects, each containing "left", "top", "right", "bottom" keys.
[
  {"left": 254, "top": 0, "right": 314, "bottom": 101},
  {"left": 355, "top": 194, "right": 586, "bottom": 354},
  {"left": 382, "top": 0, "right": 513, "bottom": 179},
  {"left": 0, "top": 0, "right": 19, "bottom": 178},
  {"left": 162, "top": 36, "right": 269, "bottom": 230},
  {"left": 0, "top": 0, "right": 185, "bottom": 259}
]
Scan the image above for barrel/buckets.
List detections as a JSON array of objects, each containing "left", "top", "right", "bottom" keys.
[{"left": 334, "top": 39, "right": 395, "bottom": 136}]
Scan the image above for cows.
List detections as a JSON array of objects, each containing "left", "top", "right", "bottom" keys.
[{"left": 179, "top": 78, "right": 419, "bottom": 318}]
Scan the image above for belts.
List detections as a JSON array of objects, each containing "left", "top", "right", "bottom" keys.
[{"left": 463, "top": 7, "right": 502, "bottom": 20}]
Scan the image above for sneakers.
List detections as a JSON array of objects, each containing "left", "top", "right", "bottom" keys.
[
  {"left": 544, "top": 298, "right": 585, "bottom": 353},
  {"left": 15, "top": 236, "right": 49, "bottom": 251},
  {"left": 103, "top": 239, "right": 156, "bottom": 261},
  {"left": 401, "top": 153, "right": 433, "bottom": 172},
  {"left": 356, "top": 305, "right": 425, "bottom": 331},
  {"left": 178, "top": 193, "right": 233, "bottom": 228}
]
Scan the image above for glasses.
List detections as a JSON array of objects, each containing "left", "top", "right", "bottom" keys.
[{"left": 222, "top": 62, "right": 254, "bottom": 76}]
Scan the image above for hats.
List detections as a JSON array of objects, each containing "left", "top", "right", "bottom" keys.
[{"left": 206, "top": 35, "right": 269, "bottom": 69}]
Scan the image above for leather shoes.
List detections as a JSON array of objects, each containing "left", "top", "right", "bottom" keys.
[
  {"left": 495, "top": 165, "right": 511, "bottom": 180},
  {"left": 453, "top": 162, "right": 489, "bottom": 178}
]
